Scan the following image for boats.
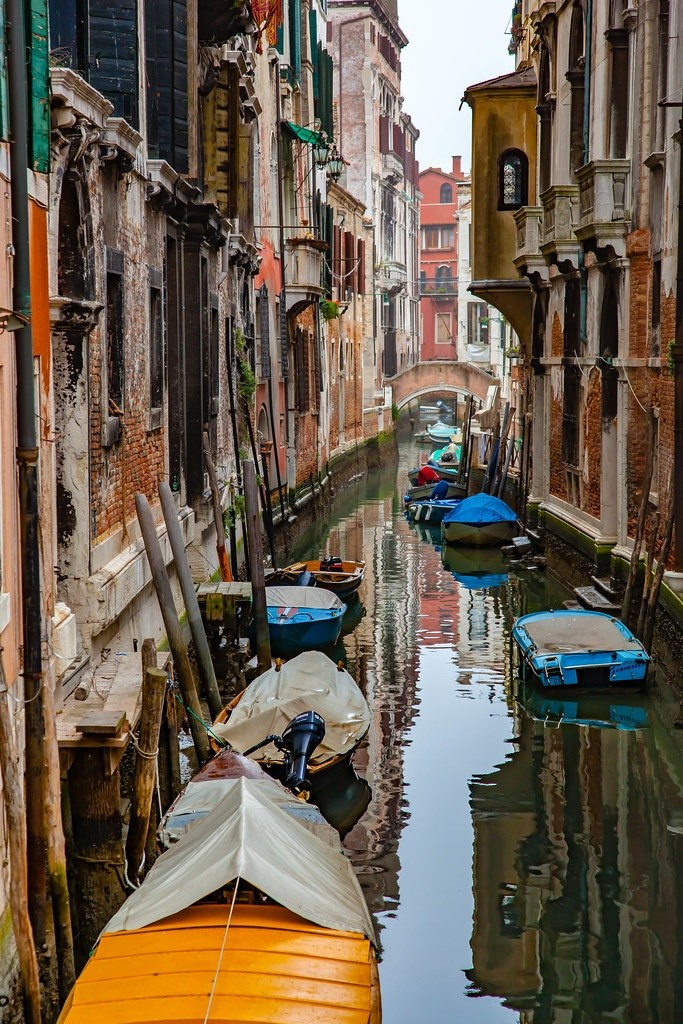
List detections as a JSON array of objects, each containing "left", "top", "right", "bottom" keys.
[
  {"left": 440, "top": 542, "right": 517, "bottom": 590},
  {"left": 56, "top": 777, "right": 384, "bottom": 1024},
  {"left": 409, "top": 522, "right": 440, "bottom": 548},
  {"left": 441, "top": 493, "right": 523, "bottom": 546},
  {"left": 404, "top": 421, "right": 463, "bottom": 525},
  {"left": 156, "top": 743, "right": 341, "bottom": 904},
  {"left": 286, "top": 556, "right": 366, "bottom": 594},
  {"left": 513, "top": 659, "right": 655, "bottom": 731},
  {"left": 248, "top": 585, "right": 346, "bottom": 659},
  {"left": 210, "top": 650, "right": 372, "bottom": 779},
  {"left": 513, "top": 610, "right": 654, "bottom": 688}
]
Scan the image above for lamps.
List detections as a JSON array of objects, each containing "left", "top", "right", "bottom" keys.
[
  {"left": 290, "top": 145, "right": 349, "bottom": 217},
  {"left": 282, "top": 122, "right": 334, "bottom": 178}
]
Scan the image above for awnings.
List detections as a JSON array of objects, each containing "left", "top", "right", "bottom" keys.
[{"left": 284, "top": 119, "right": 329, "bottom": 147}]
